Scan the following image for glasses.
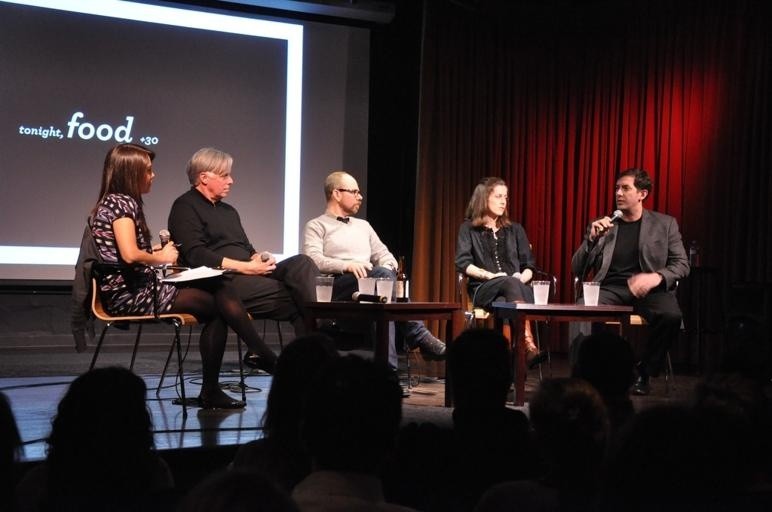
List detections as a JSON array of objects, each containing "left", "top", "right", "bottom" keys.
[{"left": 336, "top": 189, "right": 362, "bottom": 197}]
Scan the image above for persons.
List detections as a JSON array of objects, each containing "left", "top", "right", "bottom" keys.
[
  {"left": 304, "top": 172, "right": 446, "bottom": 397},
  {"left": 571, "top": 167, "right": 692, "bottom": 395},
  {"left": 177, "top": 325, "right": 772, "bottom": 511},
  {"left": 168, "top": 147, "right": 321, "bottom": 338},
  {"left": 453, "top": 177, "right": 548, "bottom": 401},
  {"left": 1, "top": 391, "right": 25, "bottom": 512},
  {"left": 15, "top": 368, "right": 178, "bottom": 511},
  {"left": 91, "top": 143, "right": 278, "bottom": 409}
]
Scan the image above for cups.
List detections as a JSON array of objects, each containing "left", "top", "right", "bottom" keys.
[
  {"left": 581, "top": 282, "right": 601, "bottom": 307},
  {"left": 531, "top": 280, "right": 550, "bottom": 305},
  {"left": 315, "top": 278, "right": 393, "bottom": 302}
]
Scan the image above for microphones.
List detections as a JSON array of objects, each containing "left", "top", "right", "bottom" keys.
[
  {"left": 593, "top": 208, "right": 623, "bottom": 241},
  {"left": 350, "top": 291, "right": 388, "bottom": 305},
  {"left": 157, "top": 228, "right": 171, "bottom": 280},
  {"left": 258, "top": 250, "right": 270, "bottom": 260},
  {"left": 508, "top": 269, "right": 523, "bottom": 282}
]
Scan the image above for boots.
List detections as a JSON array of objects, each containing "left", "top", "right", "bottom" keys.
[{"left": 512, "top": 300, "right": 549, "bottom": 371}]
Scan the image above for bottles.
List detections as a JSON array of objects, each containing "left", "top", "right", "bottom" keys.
[{"left": 395, "top": 256, "right": 410, "bottom": 302}]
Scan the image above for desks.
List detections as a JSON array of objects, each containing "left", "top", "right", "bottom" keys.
[
  {"left": 493, "top": 302, "right": 633, "bottom": 407},
  {"left": 303, "top": 301, "right": 464, "bottom": 409}
]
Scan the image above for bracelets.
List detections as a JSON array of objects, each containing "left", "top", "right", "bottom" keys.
[{"left": 480, "top": 269, "right": 490, "bottom": 279}]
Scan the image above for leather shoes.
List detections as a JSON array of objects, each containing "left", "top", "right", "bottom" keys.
[
  {"left": 419, "top": 331, "right": 447, "bottom": 360},
  {"left": 243, "top": 349, "right": 279, "bottom": 376},
  {"left": 402, "top": 386, "right": 410, "bottom": 396},
  {"left": 633, "top": 377, "right": 649, "bottom": 395},
  {"left": 197, "top": 394, "right": 246, "bottom": 410}
]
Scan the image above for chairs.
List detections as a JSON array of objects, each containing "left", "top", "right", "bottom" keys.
[
  {"left": 458, "top": 270, "right": 552, "bottom": 384},
  {"left": 574, "top": 277, "right": 680, "bottom": 398},
  {"left": 153, "top": 244, "right": 255, "bottom": 402},
  {"left": 86, "top": 277, "right": 211, "bottom": 418}
]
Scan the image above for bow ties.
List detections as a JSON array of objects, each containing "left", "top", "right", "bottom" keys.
[{"left": 337, "top": 217, "right": 349, "bottom": 224}]
